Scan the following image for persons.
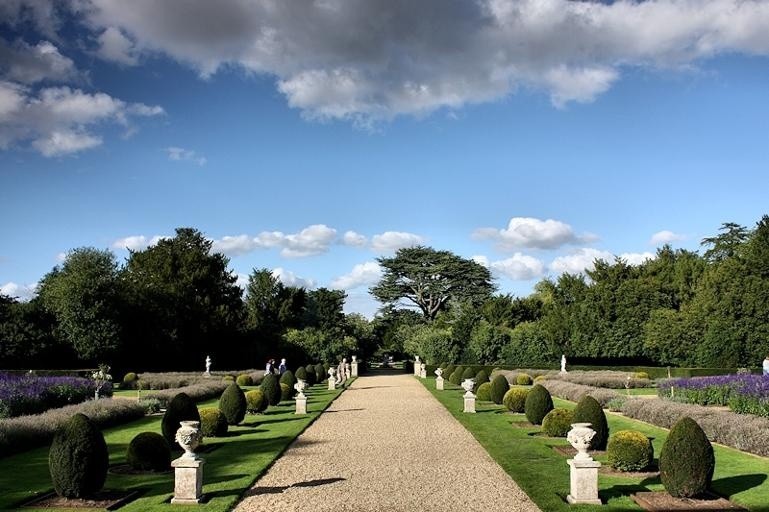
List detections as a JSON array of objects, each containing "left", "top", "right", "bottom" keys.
[
  {"left": 264, "top": 359, "right": 276, "bottom": 377},
  {"left": 205, "top": 355, "right": 212, "bottom": 372},
  {"left": 335, "top": 361, "right": 342, "bottom": 385},
  {"left": 278, "top": 358, "right": 287, "bottom": 374},
  {"left": 561, "top": 354, "right": 568, "bottom": 372},
  {"left": 336, "top": 358, "right": 348, "bottom": 389}
]
